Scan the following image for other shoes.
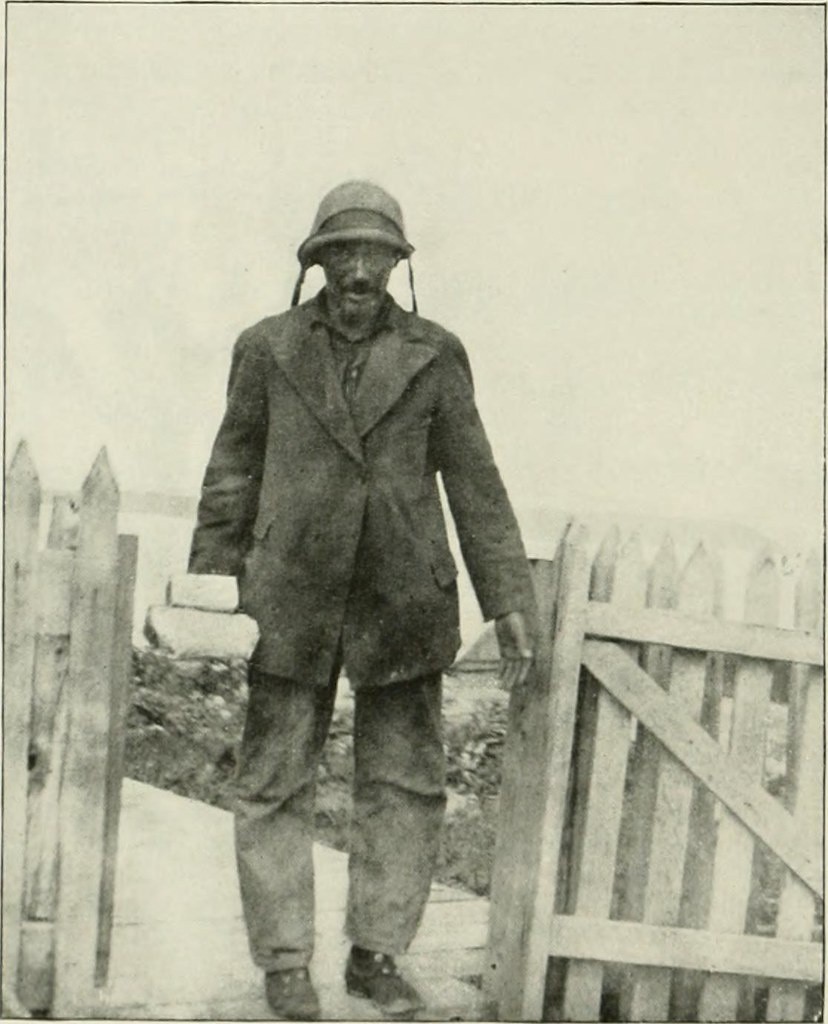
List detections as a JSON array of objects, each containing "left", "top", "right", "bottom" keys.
[
  {"left": 345, "top": 944, "right": 426, "bottom": 1016},
  {"left": 265, "top": 967, "right": 320, "bottom": 1020}
]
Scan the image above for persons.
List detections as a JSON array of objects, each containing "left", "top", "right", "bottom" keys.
[{"left": 189, "top": 181, "right": 537, "bottom": 1019}]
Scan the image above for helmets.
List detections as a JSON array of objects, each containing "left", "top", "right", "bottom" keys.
[{"left": 297, "top": 181, "right": 416, "bottom": 265}]
